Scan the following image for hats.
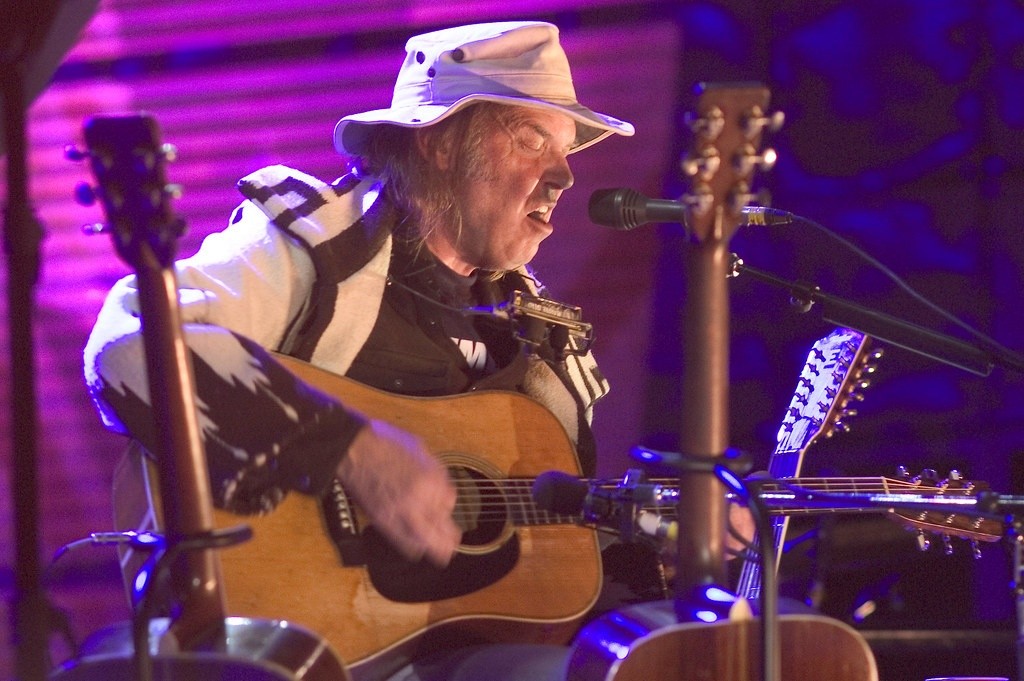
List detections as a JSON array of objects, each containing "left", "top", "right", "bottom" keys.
[{"left": 332, "top": 19, "right": 636, "bottom": 159}]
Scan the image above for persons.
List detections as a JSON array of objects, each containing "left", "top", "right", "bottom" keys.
[{"left": 82, "top": 19, "right": 760, "bottom": 680}]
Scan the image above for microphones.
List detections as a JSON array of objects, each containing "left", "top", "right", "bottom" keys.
[
  {"left": 589, "top": 187, "right": 792, "bottom": 230},
  {"left": 532, "top": 471, "right": 680, "bottom": 540}
]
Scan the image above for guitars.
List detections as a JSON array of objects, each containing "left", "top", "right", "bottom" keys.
[
  {"left": 566, "top": 78, "right": 881, "bottom": 680},
  {"left": 111, "top": 345, "right": 1008, "bottom": 681},
  {"left": 36, "top": 111, "right": 350, "bottom": 681}
]
